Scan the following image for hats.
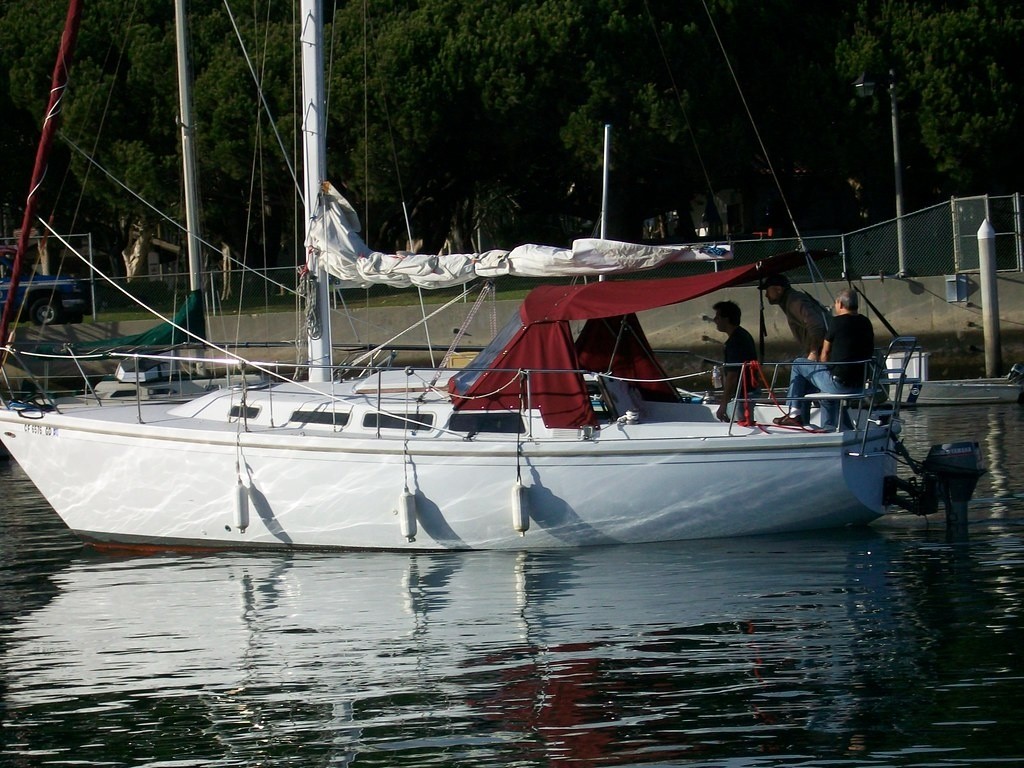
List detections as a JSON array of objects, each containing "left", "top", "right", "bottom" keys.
[{"left": 757, "top": 274, "right": 791, "bottom": 289}]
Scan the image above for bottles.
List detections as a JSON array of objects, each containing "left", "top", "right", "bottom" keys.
[{"left": 713, "top": 366, "right": 722, "bottom": 389}]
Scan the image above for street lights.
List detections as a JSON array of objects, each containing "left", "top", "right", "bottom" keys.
[{"left": 855, "top": 69, "right": 907, "bottom": 279}]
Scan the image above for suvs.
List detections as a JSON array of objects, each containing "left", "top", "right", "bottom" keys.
[{"left": 0, "top": 248, "right": 89, "bottom": 325}]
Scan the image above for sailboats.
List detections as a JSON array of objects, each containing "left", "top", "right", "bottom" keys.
[
  {"left": 1, "top": 1, "right": 300, "bottom": 400},
  {"left": 0, "top": 1, "right": 985, "bottom": 554}
]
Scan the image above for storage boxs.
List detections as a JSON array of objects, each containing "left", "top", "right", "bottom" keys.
[{"left": 883, "top": 352, "right": 928, "bottom": 384}]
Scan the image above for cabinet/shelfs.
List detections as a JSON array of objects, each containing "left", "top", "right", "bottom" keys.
[{"left": 447, "top": 352, "right": 478, "bottom": 368}]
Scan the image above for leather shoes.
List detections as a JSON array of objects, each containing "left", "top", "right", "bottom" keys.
[{"left": 773, "top": 414, "right": 802, "bottom": 426}]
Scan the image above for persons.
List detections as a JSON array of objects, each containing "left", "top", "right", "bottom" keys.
[
  {"left": 711, "top": 301, "right": 762, "bottom": 422},
  {"left": 772, "top": 286, "right": 875, "bottom": 431},
  {"left": 756, "top": 273, "right": 832, "bottom": 359}
]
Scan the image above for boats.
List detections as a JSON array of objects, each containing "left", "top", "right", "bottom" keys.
[{"left": 581, "top": 334, "right": 1023, "bottom": 413}]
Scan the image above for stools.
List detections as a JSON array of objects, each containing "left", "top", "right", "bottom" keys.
[{"left": 804, "top": 388, "right": 882, "bottom": 431}]
